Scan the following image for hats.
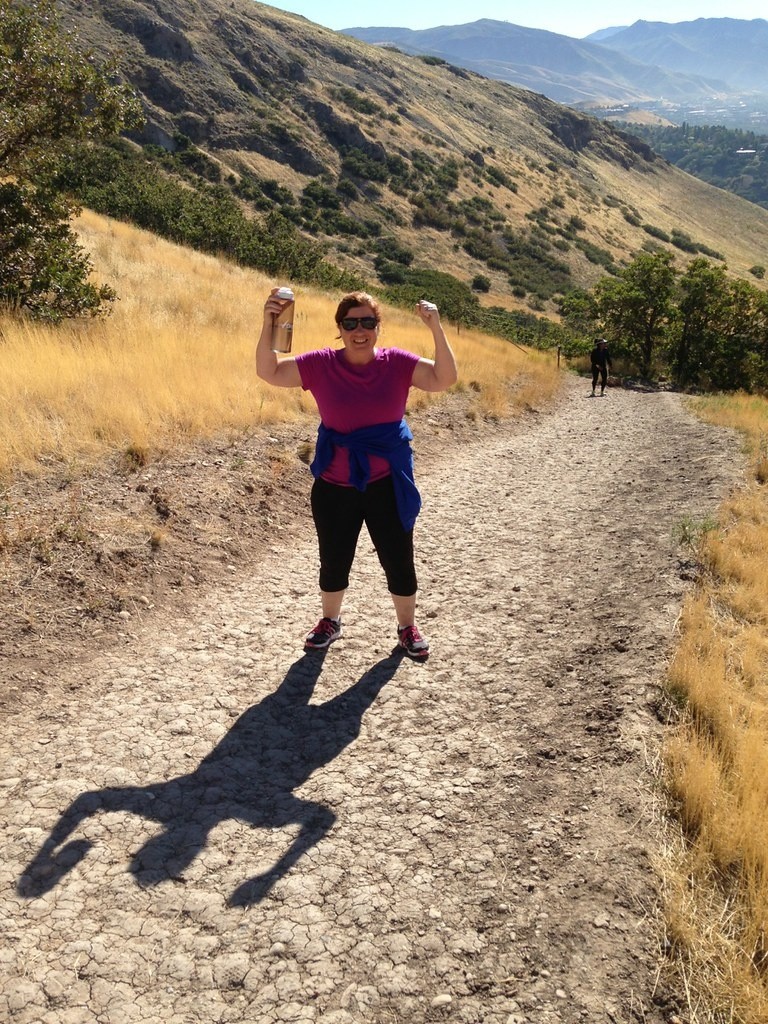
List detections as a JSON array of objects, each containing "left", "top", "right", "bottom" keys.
[{"left": 597, "top": 339, "right": 608, "bottom": 344}]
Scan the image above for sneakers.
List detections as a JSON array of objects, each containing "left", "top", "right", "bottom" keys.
[
  {"left": 397, "top": 624, "right": 431, "bottom": 657},
  {"left": 305, "top": 616, "right": 343, "bottom": 649}
]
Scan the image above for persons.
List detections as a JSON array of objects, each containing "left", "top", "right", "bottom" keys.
[
  {"left": 590, "top": 338, "right": 612, "bottom": 397},
  {"left": 255, "top": 286, "right": 458, "bottom": 657}
]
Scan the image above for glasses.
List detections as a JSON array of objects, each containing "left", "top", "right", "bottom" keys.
[{"left": 339, "top": 317, "right": 378, "bottom": 331}]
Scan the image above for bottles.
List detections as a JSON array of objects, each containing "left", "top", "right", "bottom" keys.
[{"left": 271, "top": 287, "right": 294, "bottom": 353}]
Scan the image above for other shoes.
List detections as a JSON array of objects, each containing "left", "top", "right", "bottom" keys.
[
  {"left": 592, "top": 390, "right": 596, "bottom": 396},
  {"left": 601, "top": 391, "right": 606, "bottom": 396}
]
